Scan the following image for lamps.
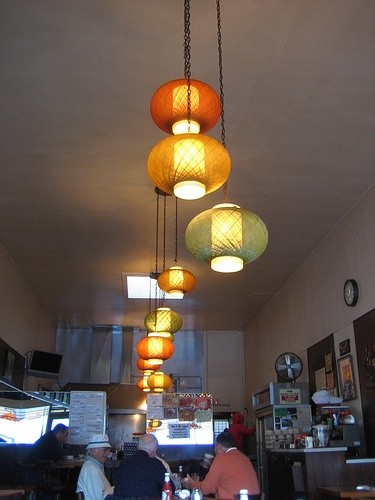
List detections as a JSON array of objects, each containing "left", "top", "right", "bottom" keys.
[{"left": 134, "top": 0, "right": 268, "bottom": 392}]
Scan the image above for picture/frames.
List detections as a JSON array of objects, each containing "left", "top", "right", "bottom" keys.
[{"left": 324, "top": 338, "right": 357, "bottom": 402}]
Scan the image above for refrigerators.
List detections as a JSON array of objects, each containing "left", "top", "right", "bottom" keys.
[{"left": 251, "top": 382, "right": 313, "bottom": 500}]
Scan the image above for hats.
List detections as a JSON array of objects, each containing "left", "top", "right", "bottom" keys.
[{"left": 85, "top": 434, "right": 112, "bottom": 449}]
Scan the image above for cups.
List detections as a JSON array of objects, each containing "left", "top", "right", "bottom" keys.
[
  {"left": 203, "top": 453, "right": 214, "bottom": 468},
  {"left": 305, "top": 436, "right": 313, "bottom": 448},
  {"left": 190, "top": 473, "right": 200, "bottom": 482}
]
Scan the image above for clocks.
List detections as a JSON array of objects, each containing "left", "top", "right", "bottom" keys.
[{"left": 344, "top": 279, "right": 359, "bottom": 306}]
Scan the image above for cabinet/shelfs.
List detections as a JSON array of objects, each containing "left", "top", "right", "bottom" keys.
[{"left": 269, "top": 445, "right": 349, "bottom": 500}]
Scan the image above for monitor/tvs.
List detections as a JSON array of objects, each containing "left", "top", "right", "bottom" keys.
[{"left": 27, "top": 350, "right": 63, "bottom": 378}]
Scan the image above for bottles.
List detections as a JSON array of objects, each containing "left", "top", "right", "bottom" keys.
[
  {"left": 162, "top": 473, "right": 173, "bottom": 500},
  {"left": 191, "top": 487, "right": 203, "bottom": 500},
  {"left": 240, "top": 489, "right": 248, "bottom": 500},
  {"left": 178, "top": 466, "right": 182, "bottom": 478}
]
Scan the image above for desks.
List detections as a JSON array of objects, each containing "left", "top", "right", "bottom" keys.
[{"left": 318, "top": 486, "right": 375, "bottom": 500}]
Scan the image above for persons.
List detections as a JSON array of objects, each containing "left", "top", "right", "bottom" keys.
[
  {"left": 188, "top": 428, "right": 262, "bottom": 500},
  {"left": 76, "top": 433, "right": 115, "bottom": 500},
  {"left": 114, "top": 432, "right": 175, "bottom": 500},
  {"left": 18, "top": 423, "right": 73, "bottom": 500},
  {"left": 228, "top": 413, "right": 256, "bottom": 457}
]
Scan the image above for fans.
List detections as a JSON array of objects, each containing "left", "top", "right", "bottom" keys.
[{"left": 275, "top": 352, "right": 303, "bottom": 382}]
